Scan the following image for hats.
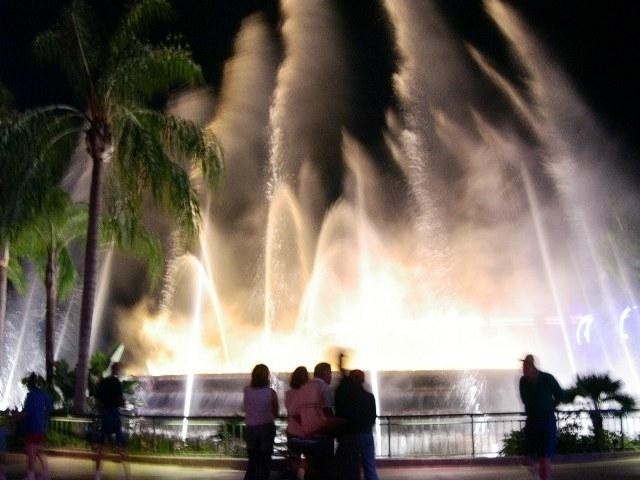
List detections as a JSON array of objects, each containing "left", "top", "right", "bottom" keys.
[{"left": 520, "top": 354, "right": 535, "bottom": 362}]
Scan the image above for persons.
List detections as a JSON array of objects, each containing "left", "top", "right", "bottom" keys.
[
  {"left": 0, "top": 371, "right": 52, "bottom": 480},
  {"left": 242, "top": 363, "right": 379, "bottom": 480},
  {"left": 92, "top": 362, "right": 133, "bottom": 480},
  {"left": 518, "top": 353, "right": 564, "bottom": 480}
]
